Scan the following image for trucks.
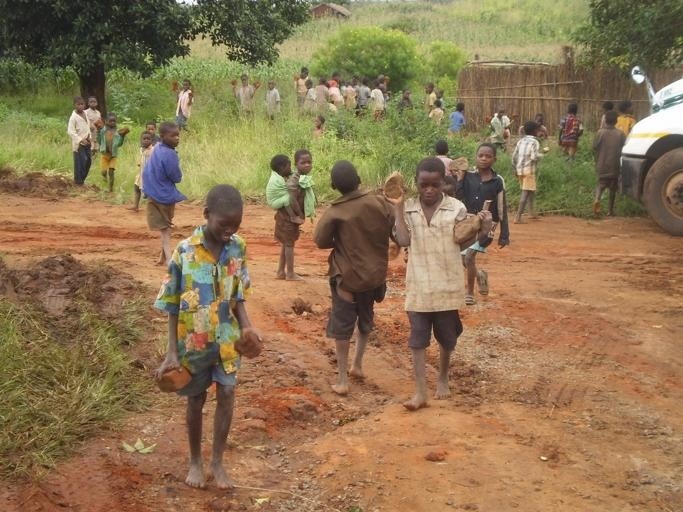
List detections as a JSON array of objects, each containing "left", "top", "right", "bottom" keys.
[{"left": 617, "top": 67, "right": 682, "bottom": 234}]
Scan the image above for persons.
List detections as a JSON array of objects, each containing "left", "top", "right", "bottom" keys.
[
  {"left": 232, "top": 74, "right": 260, "bottom": 117},
  {"left": 295, "top": 65, "right": 444, "bottom": 141},
  {"left": 382, "top": 156, "right": 494, "bottom": 411},
  {"left": 487, "top": 104, "right": 547, "bottom": 150},
  {"left": 85, "top": 97, "right": 102, "bottom": 154},
  {"left": 146, "top": 122, "right": 160, "bottom": 145},
  {"left": 141, "top": 120, "right": 188, "bottom": 265},
  {"left": 264, "top": 79, "right": 280, "bottom": 119},
  {"left": 449, "top": 144, "right": 511, "bottom": 306},
  {"left": 273, "top": 148, "right": 313, "bottom": 282},
  {"left": 265, "top": 155, "right": 304, "bottom": 226},
  {"left": 511, "top": 122, "right": 549, "bottom": 224},
  {"left": 599, "top": 100, "right": 614, "bottom": 129},
  {"left": 615, "top": 100, "right": 637, "bottom": 137},
  {"left": 435, "top": 141, "right": 454, "bottom": 176},
  {"left": 69, "top": 96, "right": 93, "bottom": 184},
  {"left": 125, "top": 131, "right": 153, "bottom": 211},
  {"left": 314, "top": 160, "right": 395, "bottom": 394},
  {"left": 151, "top": 184, "right": 268, "bottom": 489},
  {"left": 592, "top": 111, "right": 627, "bottom": 217},
  {"left": 448, "top": 102, "right": 465, "bottom": 137},
  {"left": 557, "top": 103, "right": 585, "bottom": 159},
  {"left": 172, "top": 79, "right": 194, "bottom": 134},
  {"left": 95, "top": 113, "right": 125, "bottom": 190}
]
